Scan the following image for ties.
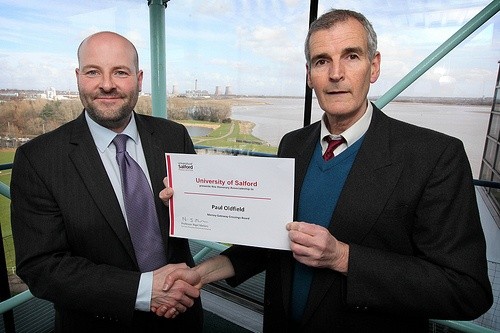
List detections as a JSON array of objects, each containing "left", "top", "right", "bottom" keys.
[
  {"left": 111, "top": 134, "right": 168, "bottom": 274},
  {"left": 322, "top": 136, "right": 346, "bottom": 161}
]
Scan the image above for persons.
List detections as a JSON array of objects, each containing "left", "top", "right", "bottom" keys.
[
  {"left": 151, "top": 9, "right": 494, "bottom": 333},
  {"left": 10, "top": 32, "right": 202, "bottom": 333}
]
